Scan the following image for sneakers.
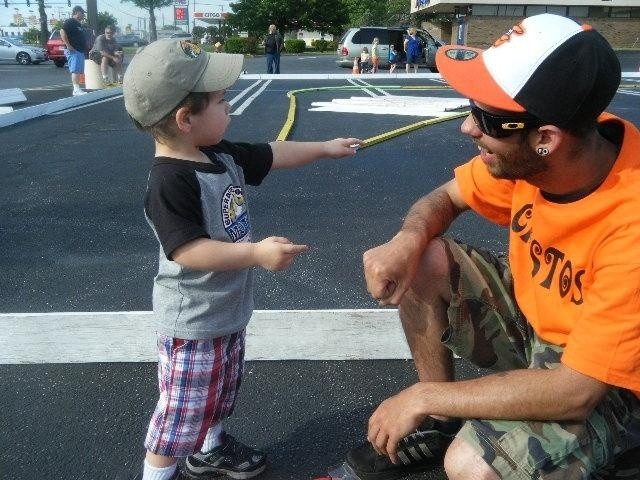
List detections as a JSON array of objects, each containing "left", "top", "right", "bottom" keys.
[
  {"left": 345, "top": 429, "right": 458, "bottom": 478},
  {"left": 73, "top": 90, "right": 87, "bottom": 96},
  {"left": 186, "top": 431, "right": 267, "bottom": 479}
]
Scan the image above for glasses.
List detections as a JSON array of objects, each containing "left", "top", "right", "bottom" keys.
[{"left": 468, "top": 97, "right": 540, "bottom": 138}]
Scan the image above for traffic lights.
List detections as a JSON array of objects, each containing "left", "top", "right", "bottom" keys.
[
  {"left": 26, "top": 0, "right": 30, "bottom": 7},
  {"left": 4, "top": 0, "right": 8, "bottom": 8},
  {"left": 67, "top": 0, "right": 71, "bottom": 7}
]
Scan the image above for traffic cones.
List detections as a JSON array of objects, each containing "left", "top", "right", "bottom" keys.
[{"left": 350, "top": 55, "right": 360, "bottom": 76}]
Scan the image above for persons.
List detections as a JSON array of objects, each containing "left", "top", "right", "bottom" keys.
[
  {"left": 371, "top": 38, "right": 381, "bottom": 74},
  {"left": 345, "top": 12, "right": 640, "bottom": 479},
  {"left": 121, "top": 34, "right": 365, "bottom": 480},
  {"left": 388, "top": 43, "right": 398, "bottom": 74},
  {"left": 403, "top": 27, "right": 423, "bottom": 74},
  {"left": 261, "top": 24, "right": 285, "bottom": 74},
  {"left": 361, "top": 47, "right": 370, "bottom": 74},
  {"left": 59, "top": 5, "right": 94, "bottom": 96},
  {"left": 90, "top": 25, "right": 124, "bottom": 86}
]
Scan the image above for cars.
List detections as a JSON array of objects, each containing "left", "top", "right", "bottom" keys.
[
  {"left": 168, "top": 32, "right": 195, "bottom": 44},
  {"left": 0, "top": 35, "right": 49, "bottom": 66},
  {"left": 112, "top": 35, "right": 148, "bottom": 47}
]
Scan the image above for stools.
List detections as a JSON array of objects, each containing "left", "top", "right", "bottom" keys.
[{"left": 83, "top": 58, "right": 114, "bottom": 90}]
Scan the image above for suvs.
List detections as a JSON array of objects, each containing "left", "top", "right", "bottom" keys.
[
  {"left": 333, "top": 23, "right": 450, "bottom": 74},
  {"left": 47, "top": 28, "right": 96, "bottom": 67}
]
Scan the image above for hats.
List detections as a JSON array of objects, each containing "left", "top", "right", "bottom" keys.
[
  {"left": 73, "top": 6, "right": 86, "bottom": 12},
  {"left": 435, "top": 18, "right": 621, "bottom": 121},
  {"left": 123, "top": 38, "right": 244, "bottom": 128}
]
